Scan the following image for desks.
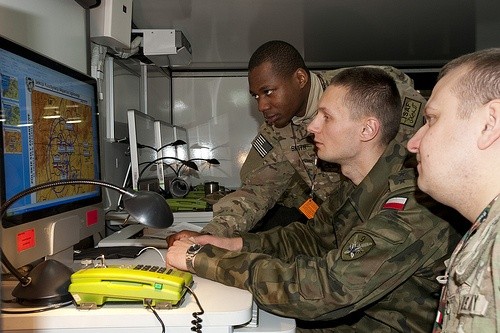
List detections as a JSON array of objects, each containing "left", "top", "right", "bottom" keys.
[{"left": 0, "top": 229, "right": 296, "bottom": 333}]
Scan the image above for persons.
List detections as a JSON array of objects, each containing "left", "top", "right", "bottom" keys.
[
  {"left": 407, "top": 48, "right": 500, "bottom": 333},
  {"left": 165, "top": 39, "right": 427, "bottom": 249},
  {"left": 166, "top": 67, "right": 473, "bottom": 333}
]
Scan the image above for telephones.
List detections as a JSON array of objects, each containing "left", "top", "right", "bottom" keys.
[
  {"left": 68, "top": 264, "right": 194, "bottom": 308},
  {"left": 166, "top": 184, "right": 207, "bottom": 212}
]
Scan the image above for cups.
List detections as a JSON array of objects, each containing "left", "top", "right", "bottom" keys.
[{"left": 204, "top": 182, "right": 219, "bottom": 195}]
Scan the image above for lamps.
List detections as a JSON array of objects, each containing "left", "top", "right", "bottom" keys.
[
  {"left": 139, "top": 140, "right": 220, "bottom": 197},
  {"left": 0, "top": 178, "right": 175, "bottom": 307}
]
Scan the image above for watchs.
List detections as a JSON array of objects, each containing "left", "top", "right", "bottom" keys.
[{"left": 186, "top": 243, "right": 205, "bottom": 274}]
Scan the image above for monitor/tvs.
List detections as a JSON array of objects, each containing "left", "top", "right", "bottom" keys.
[
  {"left": 128, "top": 111, "right": 190, "bottom": 198},
  {"left": 0, "top": 35, "right": 103, "bottom": 275}
]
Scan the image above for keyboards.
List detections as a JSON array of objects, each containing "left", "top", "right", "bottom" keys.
[{"left": 240, "top": 300, "right": 258, "bottom": 329}]
